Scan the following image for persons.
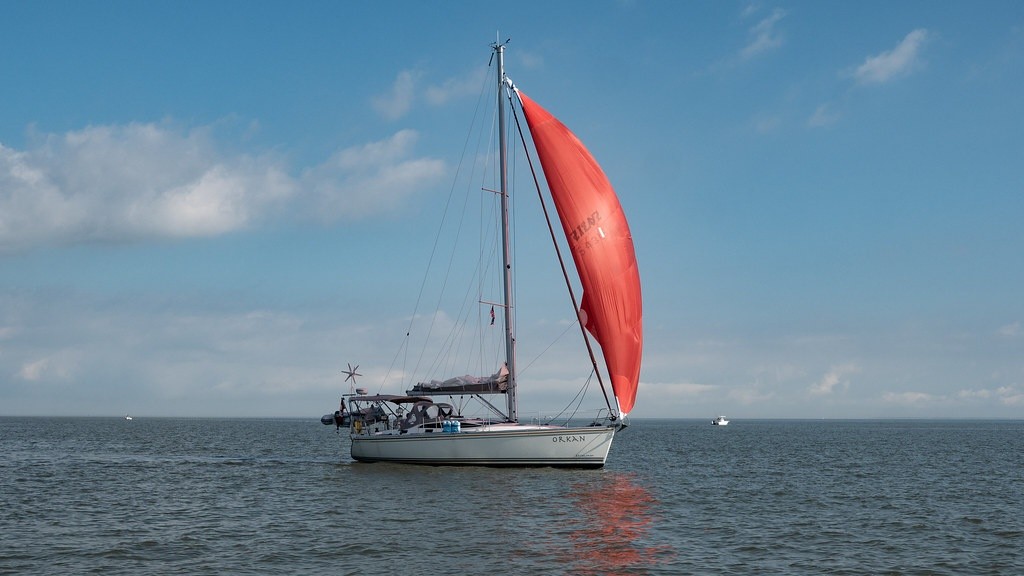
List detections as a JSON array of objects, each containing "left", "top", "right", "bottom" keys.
[{"left": 419, "top": 405, "right": 431, "bottom": 420}]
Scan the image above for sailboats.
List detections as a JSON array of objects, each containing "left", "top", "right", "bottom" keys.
[{"left": 347, "top": 31, "right": 642, "bottom": 470}]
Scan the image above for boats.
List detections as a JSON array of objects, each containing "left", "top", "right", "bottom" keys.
[{"left": 710, "top": 415, "right": 731, "bottom": 426}]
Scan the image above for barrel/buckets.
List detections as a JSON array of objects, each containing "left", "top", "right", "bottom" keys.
[
  {"left": 443, "top": 421, "right": 452, "bottom": 432},
  {"left": 452, "top": 421, "right": 460, "bottom": 434}
]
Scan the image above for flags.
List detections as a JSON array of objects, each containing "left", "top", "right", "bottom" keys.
[{"left": 338, "top": 398, "right": 345, "bottom": 421}]
